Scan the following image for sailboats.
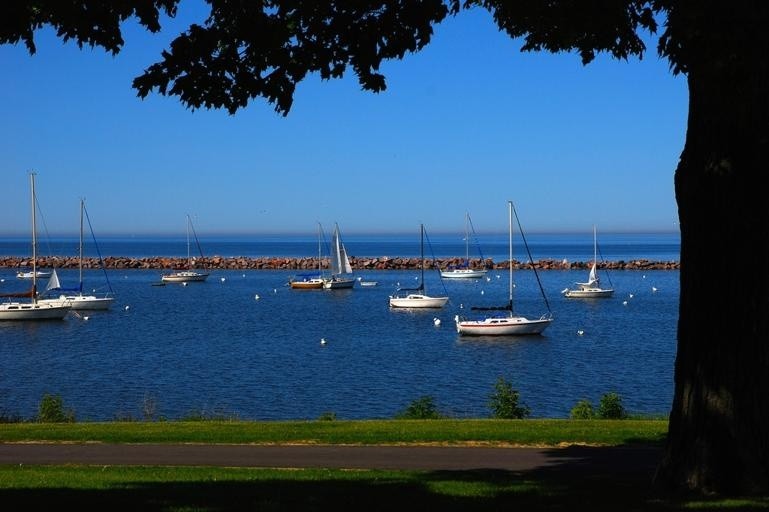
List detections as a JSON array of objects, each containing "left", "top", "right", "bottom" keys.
[
  {"left": 323, "top": 221, "right": 357, "bottom": 291},
  {"left": 0, "top": 168, "right": 117, "bottom": 323},
  {"left": 438, "top": 210, "right": 487, "bottom": 279},
  {"left": 455, "top": 198, "right": 556, "bottom": 339},
  {"left": 288, "top": 219, "right": 330, "bottom": 290},
  {"left": 387, "top": 223, "right": 454, "bottom": 310},
  {"left": 160, "top": 213, "right": 212, "bottom": 282},
  {"left": 561, "top": 221, "right": 615, "bottom": 299}
]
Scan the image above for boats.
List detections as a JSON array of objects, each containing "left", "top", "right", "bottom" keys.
[{"left": 359, "top": 281, "right": 378, "bottom": 287}]
